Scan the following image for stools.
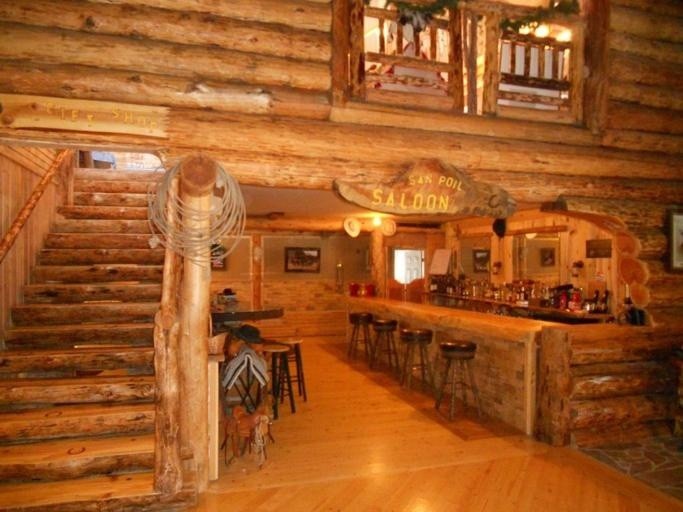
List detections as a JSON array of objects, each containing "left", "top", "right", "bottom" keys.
[
  {"left": 347, "top": 312, "right": 482, "bottom": 423},
  {"left": 219, "top": 336, "right": 307, "bottom": 450}
]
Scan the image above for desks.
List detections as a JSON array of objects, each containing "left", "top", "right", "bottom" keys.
[{"left": 209, "top": 300, "right": 284, "bottom": 332}]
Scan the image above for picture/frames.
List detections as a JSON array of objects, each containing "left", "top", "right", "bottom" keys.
[
  {"left": 473, "top": 249, "right": 490, "bottom": 273},
  {"left": 540, "top": 247, "right": 555, "bottom": 267},
  {"left": 284, "top": 246, "right": 321, "bottom": 272},
  {"left": 210, "top": 247, "right": 226, "bottom": 271},
  {"left": 666, "top": 210, "right": 683, "bottom": 273}
]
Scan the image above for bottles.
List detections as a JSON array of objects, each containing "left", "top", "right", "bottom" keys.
[{"left": 430, "top": 272, "right": 609, "bottom": 314}]
